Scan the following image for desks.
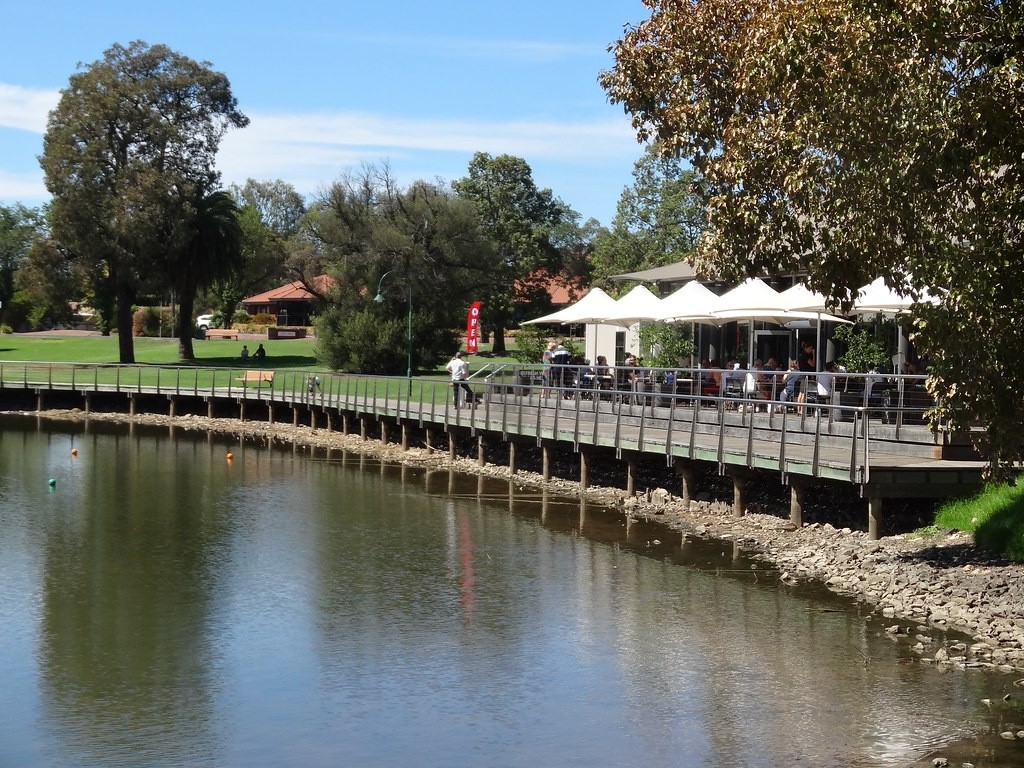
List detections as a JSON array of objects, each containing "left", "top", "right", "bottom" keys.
[{"left": 583, "top": 375, "right": 823, "bottom": 415}]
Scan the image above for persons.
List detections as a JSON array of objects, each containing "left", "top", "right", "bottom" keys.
[
  {"left": 241, "top": 345, "right": 250, "bottom": 360},
  {"left": 446, "top": 352, "right": 482, "bottom": 410},
  {"left": 253, "top": 344, "right": 266, "bottom": 361},
  {"left": 860, "top": 360, "right": 882, "bottom": 396},
  {"left": 700, "top": 357, "right": 779, "bottom": 413},
  {"left": 780, "top": 344, "right": 849, "bottom": 416},
  {"left": 905, "top": 360, "right": 922, "bottom": 384},
  {"left": 307, "top": 373, "right": 321, "bottom": 396},
  {"left": 569, "top": 352, "right": 640, "bottom": 404},
  {"left": 541, "top": 340, "right": 574, "bottom": 400}
]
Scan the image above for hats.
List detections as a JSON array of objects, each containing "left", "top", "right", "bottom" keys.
[
  {"left": 455, "top": 351, "right": 462, "bottom": 358},
  {"left": 764, "top": 358, "right": 777, "bottom": 366}
]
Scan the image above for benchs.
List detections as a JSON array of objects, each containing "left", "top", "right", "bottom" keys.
[
  {"left": 235, "top": 371, "right": 275, "bottom": 388},
  {"left": 269, "top": 328, "right": 306, "bottom": 339},
  {"left": 206, "top": 329, "right": 239, "bottom": 341}
]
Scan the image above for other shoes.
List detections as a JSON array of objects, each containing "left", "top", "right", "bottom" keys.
[
  {"left": 475, "top": 399, "right": 482, "bottom": 405},
  {"left": 567, "top": 394, "right": 572, "bottom": 397},
  {"left": 547, "top": 395, "right": 550, "bottom": 399},
  {"left": 563, "top": 393, "right": 568, "bottom": 400},
  {"left": 455, "top": 407, "right": 457, "bottom": 409},
  {"left": 541, "top": 394, "right": 544, "bottom": 398}
]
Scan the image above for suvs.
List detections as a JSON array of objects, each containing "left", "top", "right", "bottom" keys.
[{"left": 195, "top": 315, "right": 232, "bottom": 330}]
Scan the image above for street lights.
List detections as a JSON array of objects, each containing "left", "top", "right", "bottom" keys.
[{"left": 373, "top": 270, "right": 413, "bottom": 397}]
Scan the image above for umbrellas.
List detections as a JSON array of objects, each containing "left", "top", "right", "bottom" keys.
[{"left": 519, "top": 271, "right": 958, "bottom": 389}]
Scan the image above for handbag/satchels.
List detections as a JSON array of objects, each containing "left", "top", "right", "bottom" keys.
[{"left": 702, "top": 372, "right": 717, "bottom": 387}]
